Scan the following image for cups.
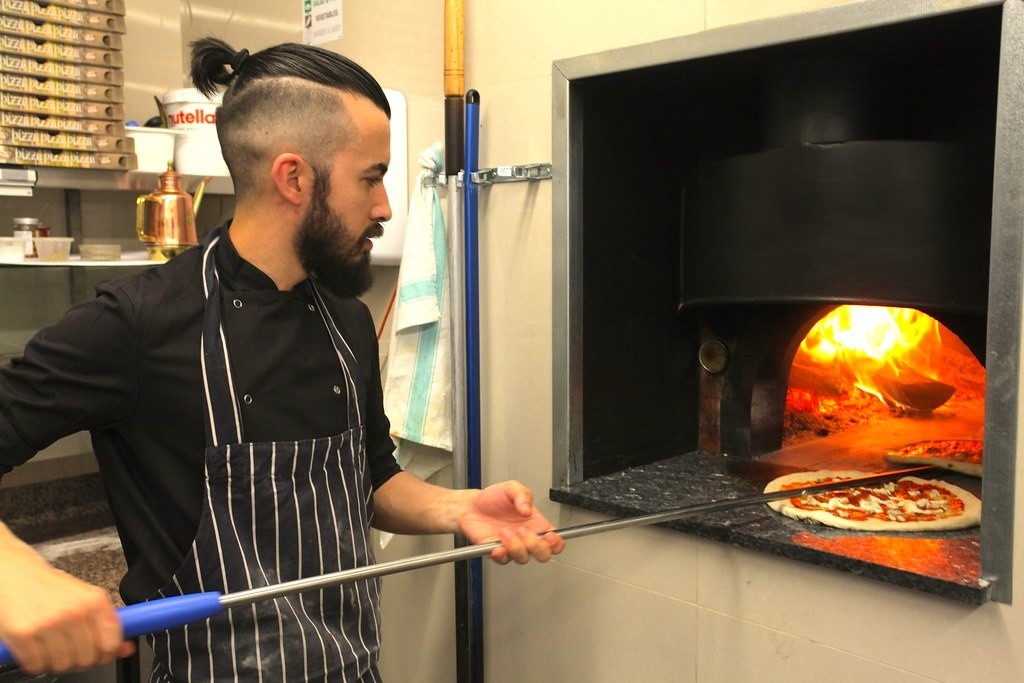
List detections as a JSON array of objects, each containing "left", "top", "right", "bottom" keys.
[{"left": 34, "top": 236, "right": 75, "bottom": 262}]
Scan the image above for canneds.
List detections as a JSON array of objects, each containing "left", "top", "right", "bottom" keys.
[{"left": 13, "top": 217, "right": 50, "bottom": 257}]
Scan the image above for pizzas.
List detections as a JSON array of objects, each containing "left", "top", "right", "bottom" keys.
[
  {"left": 885, "top": 438, "right": 983, "bottom": 478},
  {"left": 763, "top": 470, "right": 982, "bottom": 532}
]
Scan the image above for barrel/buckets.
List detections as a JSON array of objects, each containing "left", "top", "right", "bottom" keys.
[{"left": 161, "top": 89, "right": 230, "bottom": 176}]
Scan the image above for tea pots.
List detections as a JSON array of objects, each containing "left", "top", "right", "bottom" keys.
[{"left": 138, "top": 160, "right": 213, "bottom": 264}]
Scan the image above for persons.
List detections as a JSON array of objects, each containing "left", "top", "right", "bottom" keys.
[{"left": 0, "top": 33, "right": 566, "bottom": 683}]
